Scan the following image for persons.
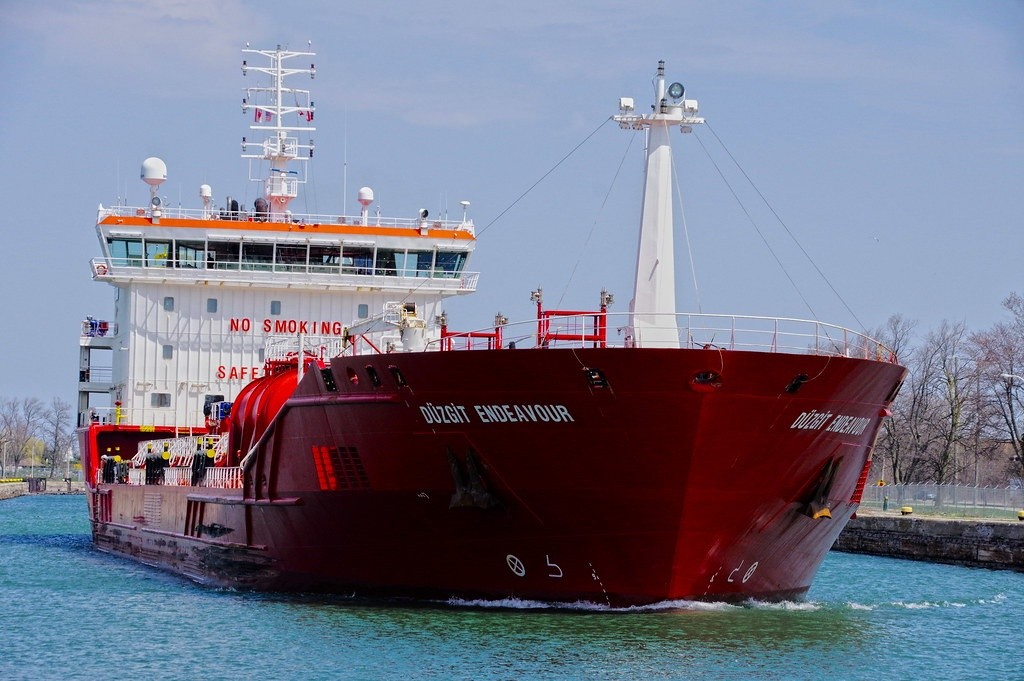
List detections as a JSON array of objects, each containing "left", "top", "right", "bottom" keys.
[{"left": 206, "top": 252, "right": 214, "bottom": 269}]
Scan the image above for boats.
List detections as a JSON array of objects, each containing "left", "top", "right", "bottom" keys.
[{"left": 74, "top": 35, "right": 908, "bottom": 617}]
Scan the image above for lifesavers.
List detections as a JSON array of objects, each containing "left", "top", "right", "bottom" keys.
[{"left": 97, "top": 265, "right": 107, "bottom": 275}]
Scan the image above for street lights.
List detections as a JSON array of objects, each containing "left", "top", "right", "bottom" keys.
[{"left": 3, "top": 439, "right": 8, "bottom": 477}]
